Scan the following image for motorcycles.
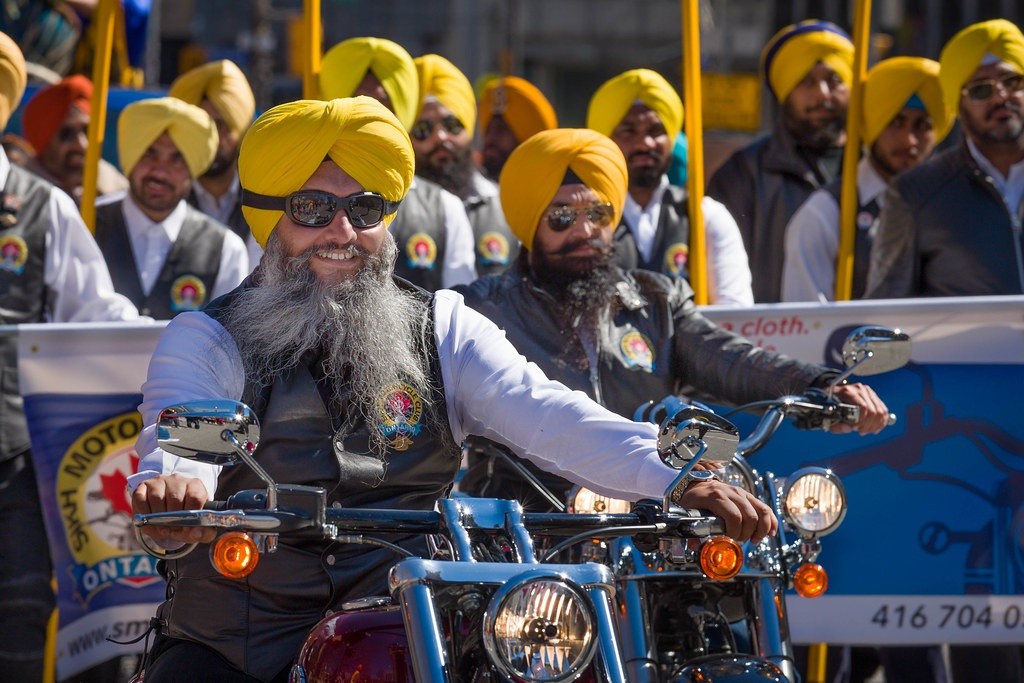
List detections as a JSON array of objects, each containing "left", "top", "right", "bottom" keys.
[
  {"left": 463, "top": 327, "right": 914, "bottom": 683},
  {"left": 133, "top": 397, "right": 744, "bottom": 683}
]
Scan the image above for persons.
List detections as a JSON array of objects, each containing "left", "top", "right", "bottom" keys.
[
  {"left": 861, "top": 17, "right": 1024, "bottom": 683},
  {"left": 80, "top": 96, "right": 249, "bottom": 325},
  {"left": 585, "top": 68, "right": 756, "bottom": 309},
  {"left": 123, "top": 93, "right": 777, "bottom": 683},
  {"left": 0, "top": 0, "right": 1024, "bottom": 323},
  {"left": 0, "top": 29, "right": 175, "bottom": 683},
  {"left": 430, "top": 127, "right": 892, "bottom": 683}
]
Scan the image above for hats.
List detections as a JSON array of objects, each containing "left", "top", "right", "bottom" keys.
[
  {"left": 23, "top": 74, "right": 94, "bottom": 157},
  {"left": 759, "top": 19, "right": 856, "bottom": 107},
  {"left": 318, "top": 36, "right": 421, "bottom": 134},
  {"left": 415, "top": 54, "right": 477, "bottom": 139},
  {"left": 238, "top": 94, "right": 416, "bottom": 254},
  {"left": 168, "top": 59, "right": 256, "bottom": 143},
  {"left": 0, "top": 29, "right": 28, "bottom": 132},
  {"left": 116, "top": 97, "right": 220, "bottom": 179},
  {"left": 478, "top": 77, "right": 557, "bottom": 144},
  {"left": 939, "top": 19, "right": 1024, "bottom": 120},
  {"left": 500, "top": 127, "right": 628, "bottom": 250},
  {"left": 860, "top": 56, "right": 955, "bottom": 159},
  {"left": 586, "top": 67, "right": 684, "bottom": 153}
]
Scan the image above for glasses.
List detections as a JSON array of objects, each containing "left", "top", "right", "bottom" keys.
[
  {"left": 962, "top": 74, "right": 1024, "bottom": 103},
  {"left": 413, "top": 115, "right": 465, "bottom": 141},
  {"left": 538, "top": 202, "right": 615, "bottom": 230},
  {"left": 241, "top": 189, "right": 405, "bottom": 230},
  {"left": 57, "top": 124, "right": 88, "bottom": 143}
]
